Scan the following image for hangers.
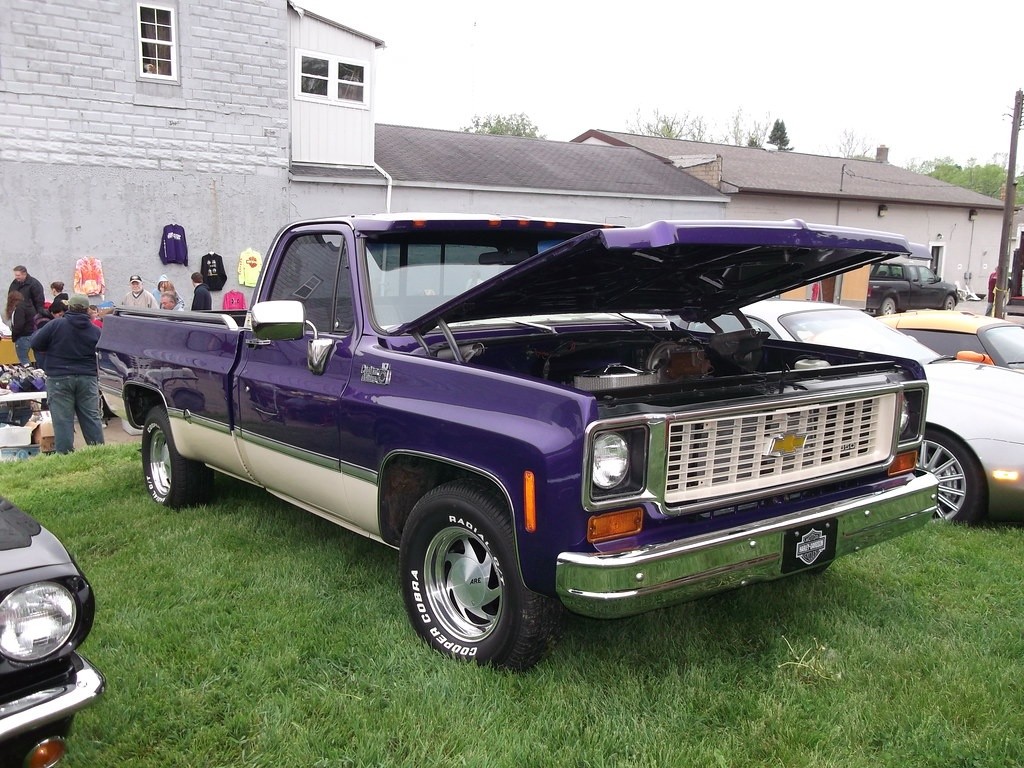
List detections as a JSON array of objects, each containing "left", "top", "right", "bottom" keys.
[
  {"left": 202, "top": 247, "right": 223, "bottom": 260},
  {"left": 164, "top": 218, "right": 184, "bottom": 230},
  {"left": 76, "top": 249, "right": 101, "bottom": 264},
  {"left": 225, "top": 284, "right": 244, "bottom": 296}
]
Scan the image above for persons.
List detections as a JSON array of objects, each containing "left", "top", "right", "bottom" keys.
[
  {"left": 191, "top": 272, "right": 212, "bottom": 310},
  {"left": 121, "top": 275, "right": 159, "bottom": 309},
  {"left": 51, "top": 281, "right": 68, "bottom": 311},
  {"left": 153, "top": 274, "right": 172, "bottom": 306},
  {"left": 5, "top": 290, "right": 36, "bottom": 367},
  {"left": 984, "top": 266, "right": 998, "bottom": 316},
  {"left": 31, "top": 294, "right": 105, "bottom": 455},
  {"left": 8, "top": 265, "right": 46, "bottom": 312},
  {"left": 89, "top": 304, "right": 103, "bottom": 328},
  {"left": 160, "top": 291, "right": 184, "bottom": 311},
  {"left": 32, "top": 301, "right": 66, "bottom": 369}
]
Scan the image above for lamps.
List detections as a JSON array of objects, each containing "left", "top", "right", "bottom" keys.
[
  {"left": 878, "top": 205, "right": 889, "bottom": 217},
  {"left": 968, "top": 209, "right": 978, "bottom": 221}
]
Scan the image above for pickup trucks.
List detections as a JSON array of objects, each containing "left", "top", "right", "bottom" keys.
[
  {"left": 93, "top": 203, "right": 943, "bottom": 672},
  {"left": 864, "top": 258, "right": 960, "bottom": 312}
]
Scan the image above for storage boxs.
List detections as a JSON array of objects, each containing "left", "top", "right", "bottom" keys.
[{"left": 0, "top": 411, "right": 56, "bottom": 462}]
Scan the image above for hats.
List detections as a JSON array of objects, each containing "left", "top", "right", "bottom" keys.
[
  {"left": 129, "top": 275, "right": 142, "bottom": 282},
  {"left": 68, "top": 294, "right": 89, "bottom": 309}
]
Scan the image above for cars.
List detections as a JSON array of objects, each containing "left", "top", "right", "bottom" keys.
[
  {"left": 660, "top": 298, "right": 1024, "bottom": 523},
  {"left": 0, "top": 499, "right": 111, "bottom": 768},
  {"left": 879, "top": 308, "right": 1024, "bottom": 369}
]
